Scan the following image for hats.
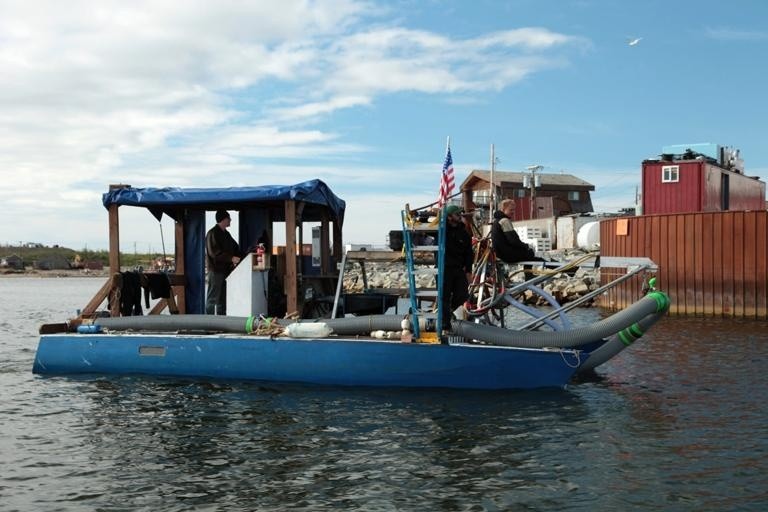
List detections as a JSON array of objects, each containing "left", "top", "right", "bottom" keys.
[
  {"left": 448, "top": 205, "right": 462, "bottom": 215},
  {"left": 216, "top": 210, "right": 229, "bottom": 223}
]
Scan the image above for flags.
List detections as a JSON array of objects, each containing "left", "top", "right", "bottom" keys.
[{"left": 437, "top": 145, "right": 456, "bottom": 209}]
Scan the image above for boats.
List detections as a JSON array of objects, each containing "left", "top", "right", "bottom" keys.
[{"left": 33, "top": 179, "right": 670, "bottom": 390}]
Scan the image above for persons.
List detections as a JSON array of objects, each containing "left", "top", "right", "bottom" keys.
[
  {"left": 491, "top": 199, "right": 540, "bottom": 282},
  {"left": 205, "top": 209, "right": 240, "bottom": 315},
  {"left": 434, "top": 205, "right": 472, "bottom": 330}
]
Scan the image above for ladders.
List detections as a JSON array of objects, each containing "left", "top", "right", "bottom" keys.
[{"left": 401, "top": 203, "right": 447, "bottom": 345}]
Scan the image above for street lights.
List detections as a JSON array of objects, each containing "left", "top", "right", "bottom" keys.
[{"left": 523, "top": 166, "right": 543, "bottom": 220}]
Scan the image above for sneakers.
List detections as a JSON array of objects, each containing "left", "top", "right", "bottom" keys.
[{"left": 441, "top": 329, "right": 449, "bottom": 335}]
